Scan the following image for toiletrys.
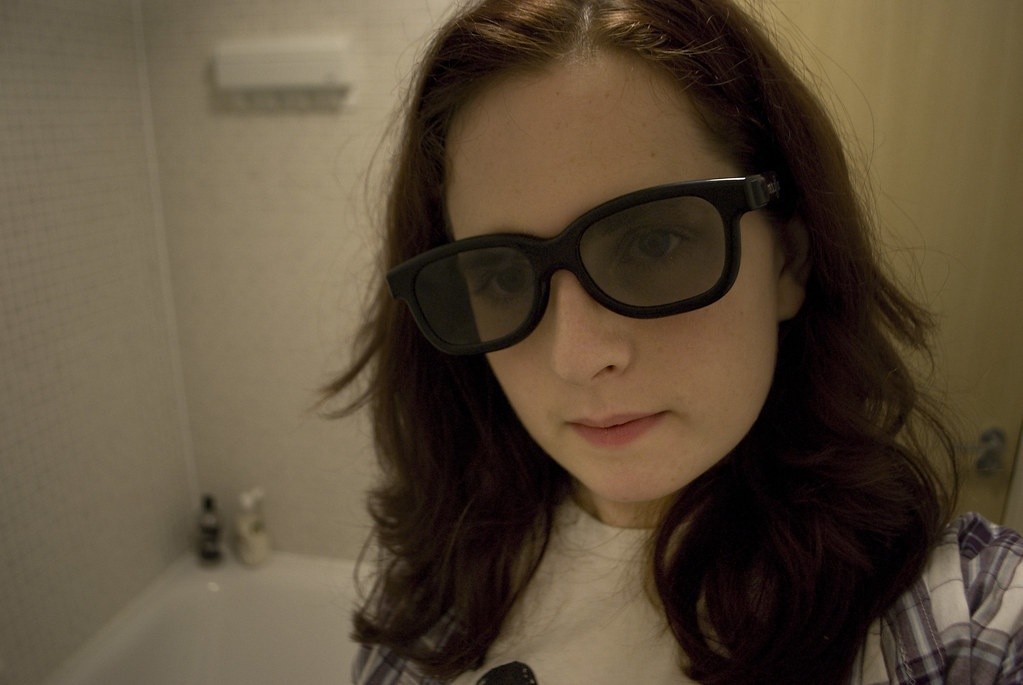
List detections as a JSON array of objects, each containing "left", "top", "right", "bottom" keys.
[
  {"left": 232, "top": 486, "right": 272, "bottom": 569},
  {"left": 196, "top": 494, "right": 224, "bottom": 570}
]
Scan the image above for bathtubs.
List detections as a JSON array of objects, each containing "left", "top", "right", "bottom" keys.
[{"left": 45, "top": 537, "right": 435, "bottom": 685}]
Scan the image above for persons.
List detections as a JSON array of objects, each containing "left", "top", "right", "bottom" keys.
[{"left": 329, "top": 1, "right": 1022, "bottom": 685}]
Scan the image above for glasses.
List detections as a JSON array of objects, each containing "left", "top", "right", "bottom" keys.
[{"left": 386, "top": 172, "right": 800, "bottom": 357}]
